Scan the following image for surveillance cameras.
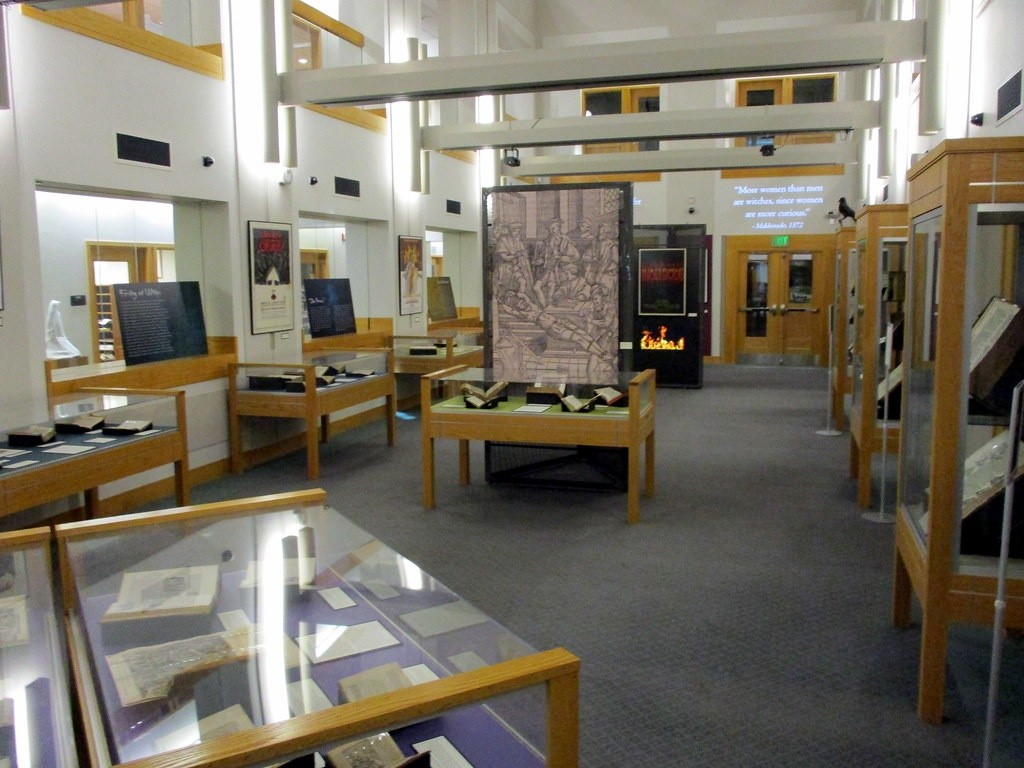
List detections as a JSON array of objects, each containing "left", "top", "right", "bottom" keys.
[
  {"left": 761, "top": 145, "right": 775, "bottom": 156},
  {"left": 505, "top": 157, "right": 521, "bottom": 167}
]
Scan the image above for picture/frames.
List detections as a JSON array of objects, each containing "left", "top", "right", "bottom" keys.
[
  {"left": 483, "top": 181, "right": 633, "bottom": 407},
  {"left": 398, "top": 235, "right": 423, "bottom": 316},
  {"left": 247, "top": 220, "right": 295, "bottom": 336},
  {"left": 638, "top": 249, "right": 687, "bottom": 316}
]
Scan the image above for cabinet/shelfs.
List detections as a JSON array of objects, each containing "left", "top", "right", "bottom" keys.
[{"left": 830, "top": 132, "right": 1024, "bottom": 724}]
